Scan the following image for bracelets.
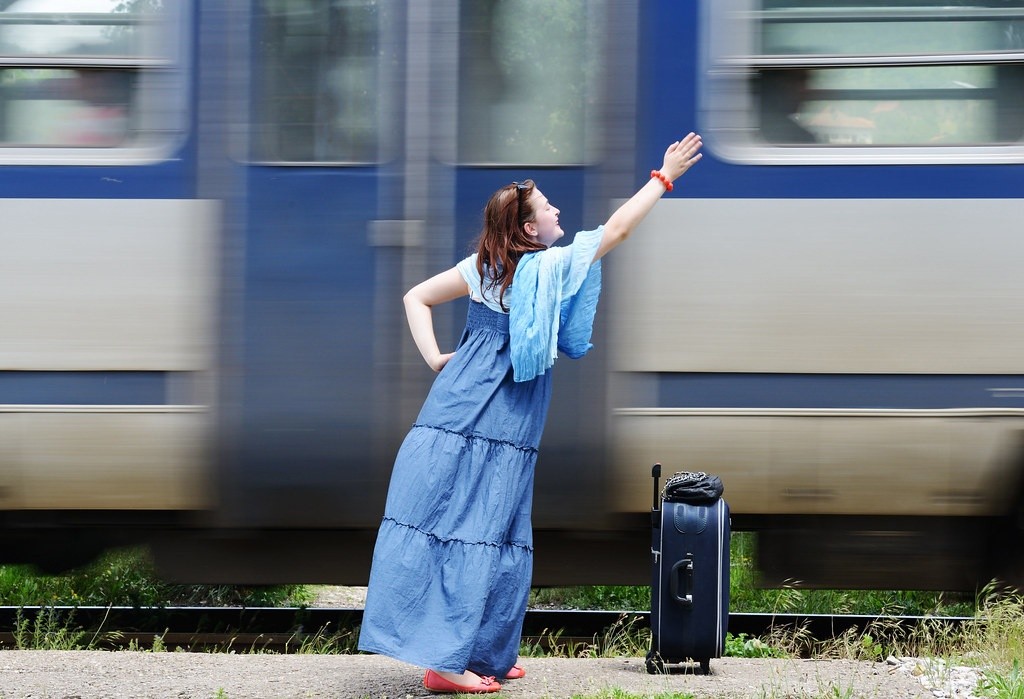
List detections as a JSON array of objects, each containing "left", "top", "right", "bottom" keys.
[{"left": 650, "top": 169, "right": 674, "bottom": 192}]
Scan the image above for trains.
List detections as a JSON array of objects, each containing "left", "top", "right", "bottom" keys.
[{"left": 0, "top": 0, "right": 1024, "bottom": 600}]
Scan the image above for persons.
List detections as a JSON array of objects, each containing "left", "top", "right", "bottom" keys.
[
  {"left": 356, "top": 129, "right": 704, "bottom": 693},
  {"left": 56, "top": 65, "right": 143, "bottom": 151},
  {"left": 748, "top": 67, "right": 818, "bottom": 144}
]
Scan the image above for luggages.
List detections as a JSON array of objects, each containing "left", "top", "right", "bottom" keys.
[{"left": 645, "top": 462, "right": 729, "bottom": 674}]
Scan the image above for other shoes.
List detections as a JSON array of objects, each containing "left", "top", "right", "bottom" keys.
[
  {"left": 499, "top": 661, "right": 526, "bottom": 679},
  {"left": 424, "top": 669, "right": 502, "bottom": 695}
]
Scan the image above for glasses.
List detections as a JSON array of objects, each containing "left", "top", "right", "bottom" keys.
[{"left": 513, "top": 180, "right": 527, "bottom": 232}]
